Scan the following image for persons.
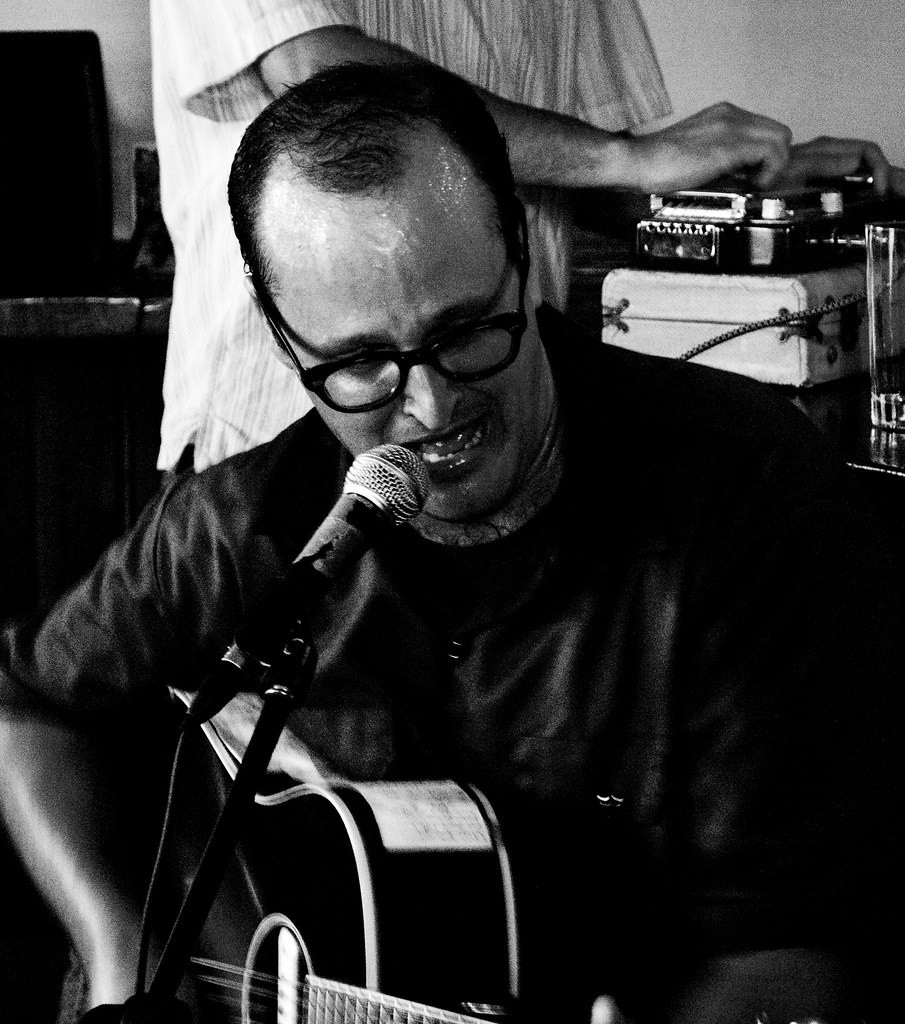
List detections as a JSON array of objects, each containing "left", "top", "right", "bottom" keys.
[
  {"left": 0, "top": 62, "right": 905, "bottom": 1024},
  {"left": 148, "top": 0, "right": 905, "bottom": 494}
]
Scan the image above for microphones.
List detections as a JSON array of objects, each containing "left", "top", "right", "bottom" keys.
[{"left": 184, "top": 445, "right": 431, "bottom": 728}]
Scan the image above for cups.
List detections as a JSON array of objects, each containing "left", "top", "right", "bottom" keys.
[{"left": 865, "top": 221, "right": 905, "bottom": 430}]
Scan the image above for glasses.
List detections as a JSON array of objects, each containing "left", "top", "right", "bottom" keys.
[{"left": 266, "top": 198, "right": 531, "bottom": 413}]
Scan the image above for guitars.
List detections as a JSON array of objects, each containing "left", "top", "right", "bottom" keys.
[{"left": 61, "top": 684, "right": 617, "bottom": 1023}]
